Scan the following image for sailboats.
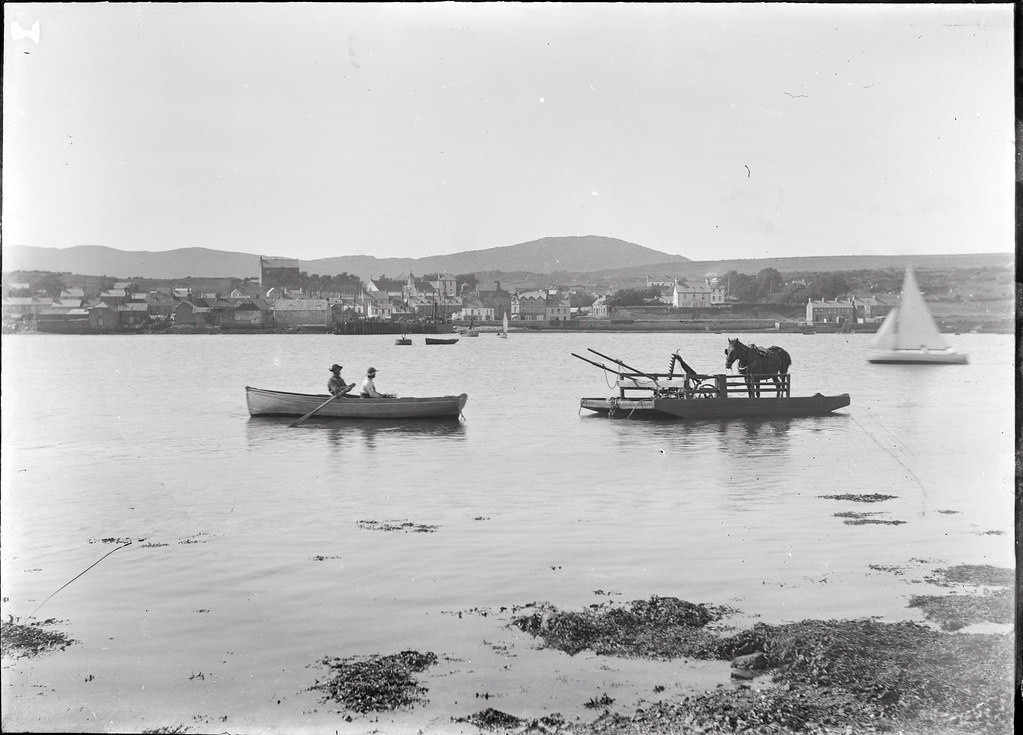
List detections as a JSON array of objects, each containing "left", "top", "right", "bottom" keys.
[
  {"left": 496, "top": 311, "right": 509, "bottom": 338},
  {"left": 866, "top": 265, "right": 969, "bottom": 365}
]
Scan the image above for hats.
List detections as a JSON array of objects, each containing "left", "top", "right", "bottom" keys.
[
  {"left": 329, "top": 364, "right": 342, "bottom": 371},
  {"left": 366, "top": 367, "right": 377, "bottom": 376}
]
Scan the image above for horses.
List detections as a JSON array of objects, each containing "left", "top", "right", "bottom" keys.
[{"left": 725, "top": 337, "right": 792, "bottom": 399}]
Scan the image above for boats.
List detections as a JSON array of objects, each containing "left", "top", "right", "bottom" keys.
[
  {"left": 571, "top": 347, "right": 850, "bottom": 420},
  {"left": 459, "top": 319, "right": 479, "bottom": 337},
  {"left": 425, "top": 337, "right": 460, "bottom": 345},
  {"left": 245, "top": 386, "right": 469, "bottom": 419},
  {"left": 394, "top": 339, "right": 413, "bottom": 345}
]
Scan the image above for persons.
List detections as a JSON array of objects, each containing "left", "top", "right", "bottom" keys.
[
  {"left": 327, "top": 364, "right": 353, "bottom": 398},
  {"left": 360, "top": 367, "right": 387, "bottom": 398}
]
